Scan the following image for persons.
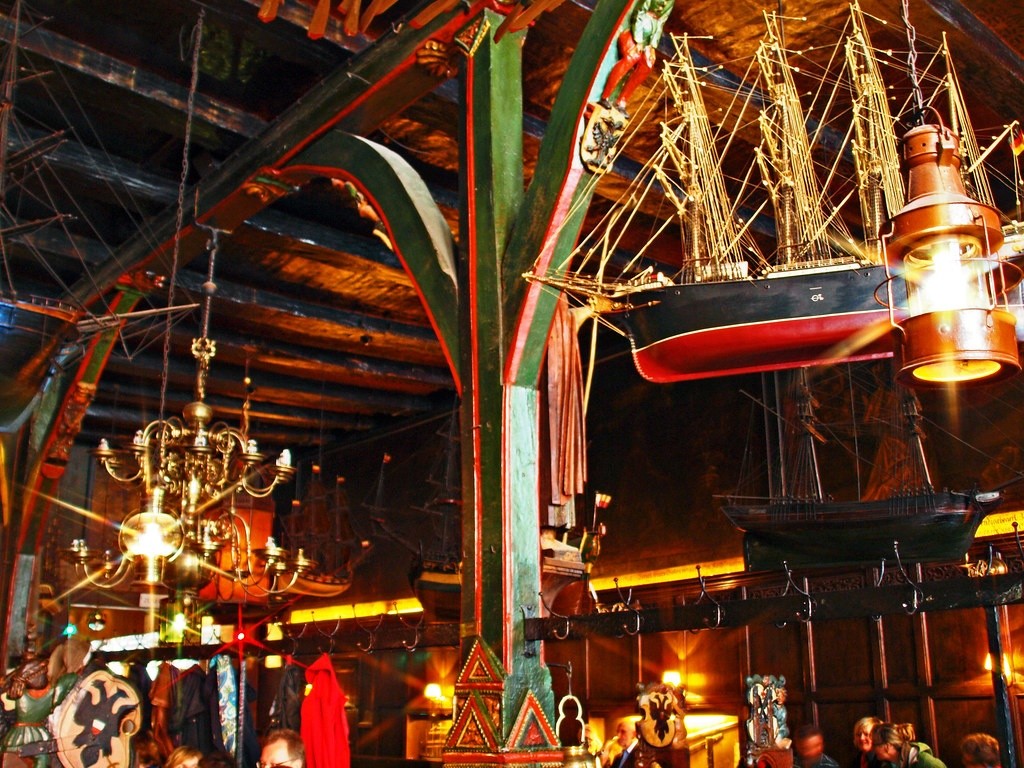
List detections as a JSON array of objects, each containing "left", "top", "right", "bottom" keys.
[
  {"left": 961, "top": 733, "right": 1001, "bottom": 768},
  {"left": 853, "top": 717, "right": 947, "bottom": 768},
  {"left": 794, "top": 726, "right": 839, "bottom": 768},
  {"left": 257, "top": 730, "right": 304, "bottom": 768}
]
